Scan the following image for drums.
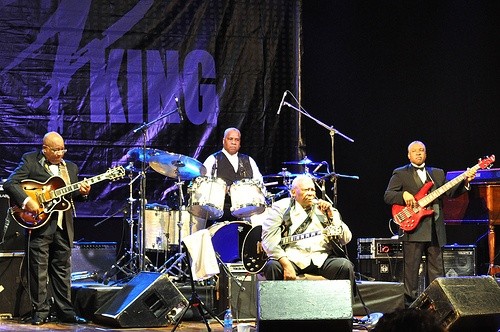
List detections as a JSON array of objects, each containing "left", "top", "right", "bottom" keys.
[
  {"left": 167, "top": 210, "right": 207, "bottom": 246},
  {"left": 186, "top": 176, "right": 227, "bottom": 220},
  {"left": 136, "top": 204, "right": 171, "bottom": 253},
  {"left": 206, "top": 221, "right": 252, "bottom": 265},
  {"left": 230, "top": 179, "right": 267, "bottom": 217}
]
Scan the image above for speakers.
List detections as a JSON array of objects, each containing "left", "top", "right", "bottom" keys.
[
  {"left": 218, "top": 263, "right": 265, "bottom": 320},
  {"left": 93, "top": 270, "right": 190, "bottom": 328},
  {"left": 70, "top": 241, "right": 118, "bottom": 281},
  {"left": 407, "top": 182, "right": 500, "bottom": 332},
  {"left": 0, "top": 182, "right": 34, "bottom": 320},
  {"left": 256, "top": 279, "right": 354, "bottom": 332}
]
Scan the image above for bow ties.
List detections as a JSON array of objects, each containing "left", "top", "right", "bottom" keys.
[
  {"left": 44, "top": 160, "right": 60, "bottom": 166},
  {"left": 413, "top": 166, "right": 425, "bottom": 171}
]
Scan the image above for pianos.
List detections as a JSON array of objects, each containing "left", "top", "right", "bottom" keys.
[{"left": 442, "top": 168, "right": 500, "bottom": 277}]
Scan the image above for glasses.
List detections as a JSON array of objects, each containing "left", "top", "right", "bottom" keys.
[{"left": 46, "top": 146, "right": 67, "bottom": 154}]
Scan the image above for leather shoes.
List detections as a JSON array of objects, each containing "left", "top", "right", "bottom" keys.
[
  {"left": 32, "top": 318, "right": 43, "bottom": 325},
  {"left": 63, "top": 315, "right": 87, "bottom": 324}
]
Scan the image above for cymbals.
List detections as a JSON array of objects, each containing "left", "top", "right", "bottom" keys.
[
  {"left": 282, "top": 160, "right": 320, "bottom": 165},
  {"left": 127, "top": 147, "right": 174, "bottom": 163},
  {"left": 148, "top": 153, "right": 207, "bottom": 181},
  {"left": 262, "top": 173, "right": 303, "bottom": 177}
]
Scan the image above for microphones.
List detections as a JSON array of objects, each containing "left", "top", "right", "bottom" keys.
[
  {"left": 175, "top": 97, "right": 183, "bottom": 120},
  {"left": 240, "top": 286, "right": 245, "bottom": 291},
  {"left": 313, "top": 161, "right": 325, "bottom": 173},
  {"left": 277, "top": 91, "right": 287, "bottom": 115}
]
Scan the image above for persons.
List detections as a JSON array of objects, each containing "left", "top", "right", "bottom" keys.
[
  {"left": 203, "top": 127, "right": 264, "bottom": 229},
  {"left": 261, "top": 174, "right": 357, "bottom": 288},
  {"left": 384, "top": 140, "right": 476, "bottom": 308},
  {"left": 3, "top": 131, "right": 92, "bottom": 325}
]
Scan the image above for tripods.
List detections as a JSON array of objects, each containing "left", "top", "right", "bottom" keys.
[{"left": 99, "top": 158, "right": 226, "bottom": 332}]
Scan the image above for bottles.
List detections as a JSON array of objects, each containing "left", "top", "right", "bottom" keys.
[{"left": 223, "top": 309, "right": 233, "bottom": 332}]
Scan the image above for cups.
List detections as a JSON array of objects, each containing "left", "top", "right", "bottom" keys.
[{"left": 237, "top": 323, "right": 251, "bottom": 332}]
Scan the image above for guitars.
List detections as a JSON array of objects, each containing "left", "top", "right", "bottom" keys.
[
  {"left": 392, "top": 155, "right": 495, "bottom": 231},
  {"left": 242, "top": 225, "right": 343, "bottom": 275},
  {"left": 9, "top": 165, "right": 125, "bottom": 230}
]
